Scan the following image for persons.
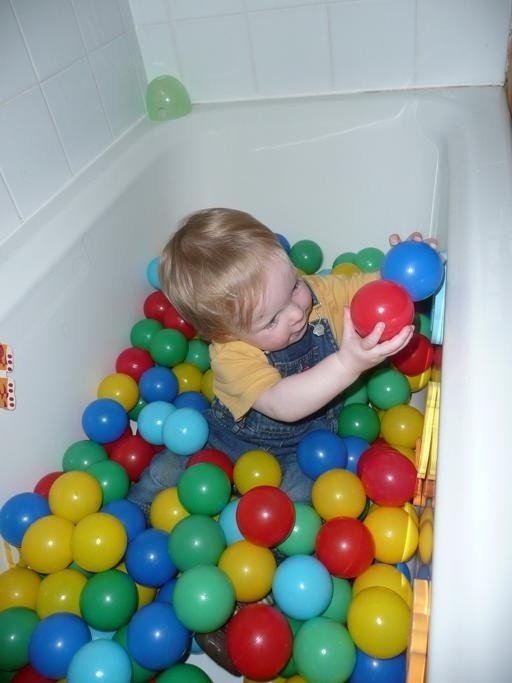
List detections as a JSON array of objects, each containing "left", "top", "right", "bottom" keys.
[{"left": 126, "top": 208, "right": 415, "bottom": 677}]
